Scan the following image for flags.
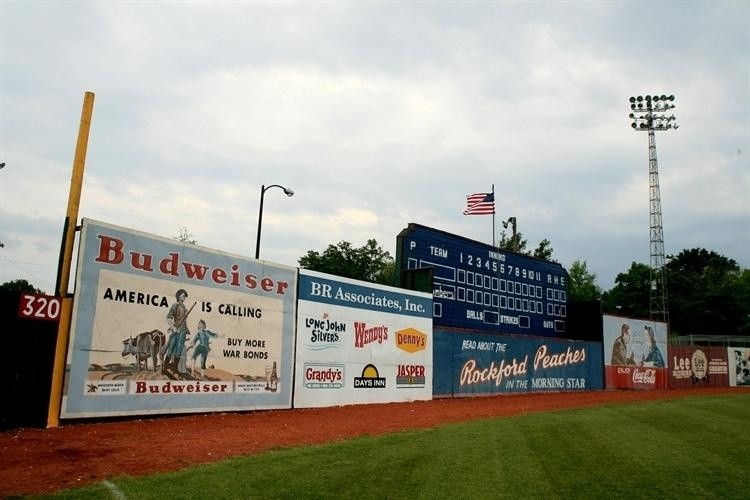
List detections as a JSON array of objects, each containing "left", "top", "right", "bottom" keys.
[{"left": 464, "top": 193, "right": 495, "bottom": 216}]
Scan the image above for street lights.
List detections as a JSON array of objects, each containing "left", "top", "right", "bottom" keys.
[
  {"left": 628, "top": 95, "right": 681, "bottom": 328},
  {"left": 254, "top": 184, "right": 293, "bottom": 261}
]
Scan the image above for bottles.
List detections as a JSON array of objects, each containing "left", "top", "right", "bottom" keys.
[{"left": 270, "top": 362, "right": 278, "bottom": 391}]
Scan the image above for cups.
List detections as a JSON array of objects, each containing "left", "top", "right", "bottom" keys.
[{"left": 265, "top": 367, "right": 272, "bottom": 387}]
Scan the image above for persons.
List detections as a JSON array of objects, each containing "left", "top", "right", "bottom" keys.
[
  {"left": 159, "top": 289, "right": 188, "bottom": 375},
  {"left": 190, "top": 317, "right": 225, "bottom": 378},
  {"left": 735, "top": 350, "right": 742, "bottom": 366},
  {"left": 641, "top": 326, "right": 664, "bottom": 368},
  {"left": 609, "top": 322, "right": 636, "bottom": 366}
]
